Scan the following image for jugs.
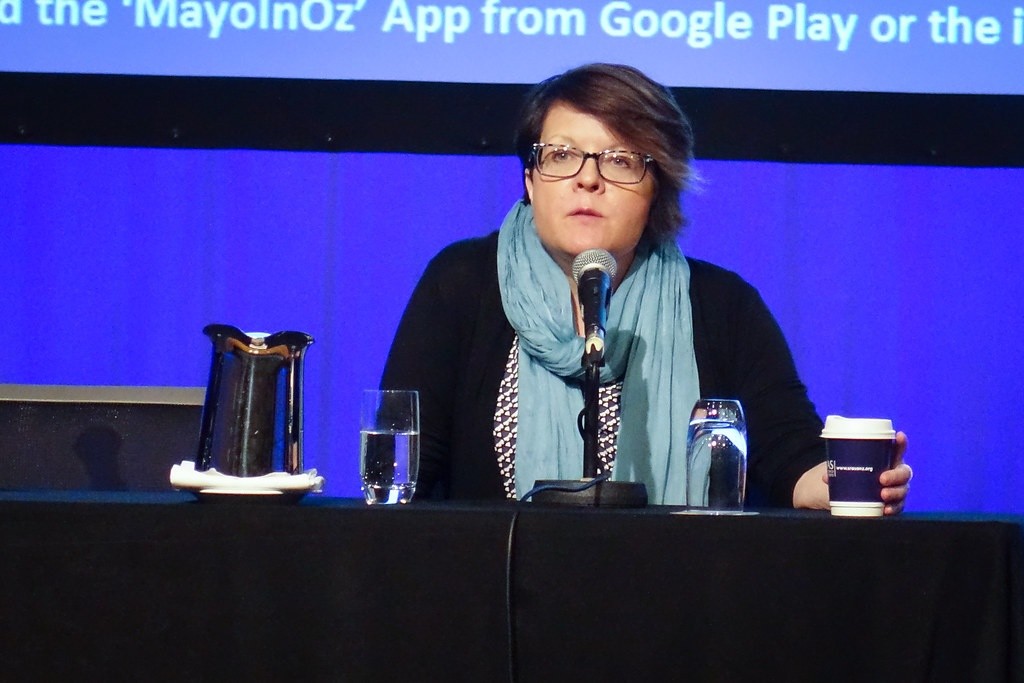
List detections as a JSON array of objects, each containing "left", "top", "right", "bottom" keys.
[{"left": 194, "top": 324, "right": 314, "bottom": 478}]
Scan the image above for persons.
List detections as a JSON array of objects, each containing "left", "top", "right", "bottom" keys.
[{"left": 364, "top": 64, "right": 912, "bottom": 513}]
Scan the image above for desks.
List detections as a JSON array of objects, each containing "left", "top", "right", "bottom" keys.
[{"left": 0, "top": 488, "right": 1024, "bottom": 683}]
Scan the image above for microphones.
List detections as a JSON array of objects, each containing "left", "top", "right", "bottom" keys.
[{"left": 572, "top": 248, "right": 617, "bottom": 364}]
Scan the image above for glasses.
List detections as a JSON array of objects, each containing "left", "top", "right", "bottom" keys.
[{"left": 532, "top": 142, "right": 654, "bottom": 185}]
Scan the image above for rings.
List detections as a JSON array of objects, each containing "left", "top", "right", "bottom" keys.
[{"left": 896, "top": 464, "right": 913, "bottom": 487}]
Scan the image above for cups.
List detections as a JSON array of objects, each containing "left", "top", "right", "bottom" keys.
[
  {"left": 686, "top": 399, "right": 747, "bottom": 514},
  {"left": 358, "top": 389, "right": 420, "bottom": 505},
  {"left": 820, "top": 415, "right": 897, "bottom": 517}
]
